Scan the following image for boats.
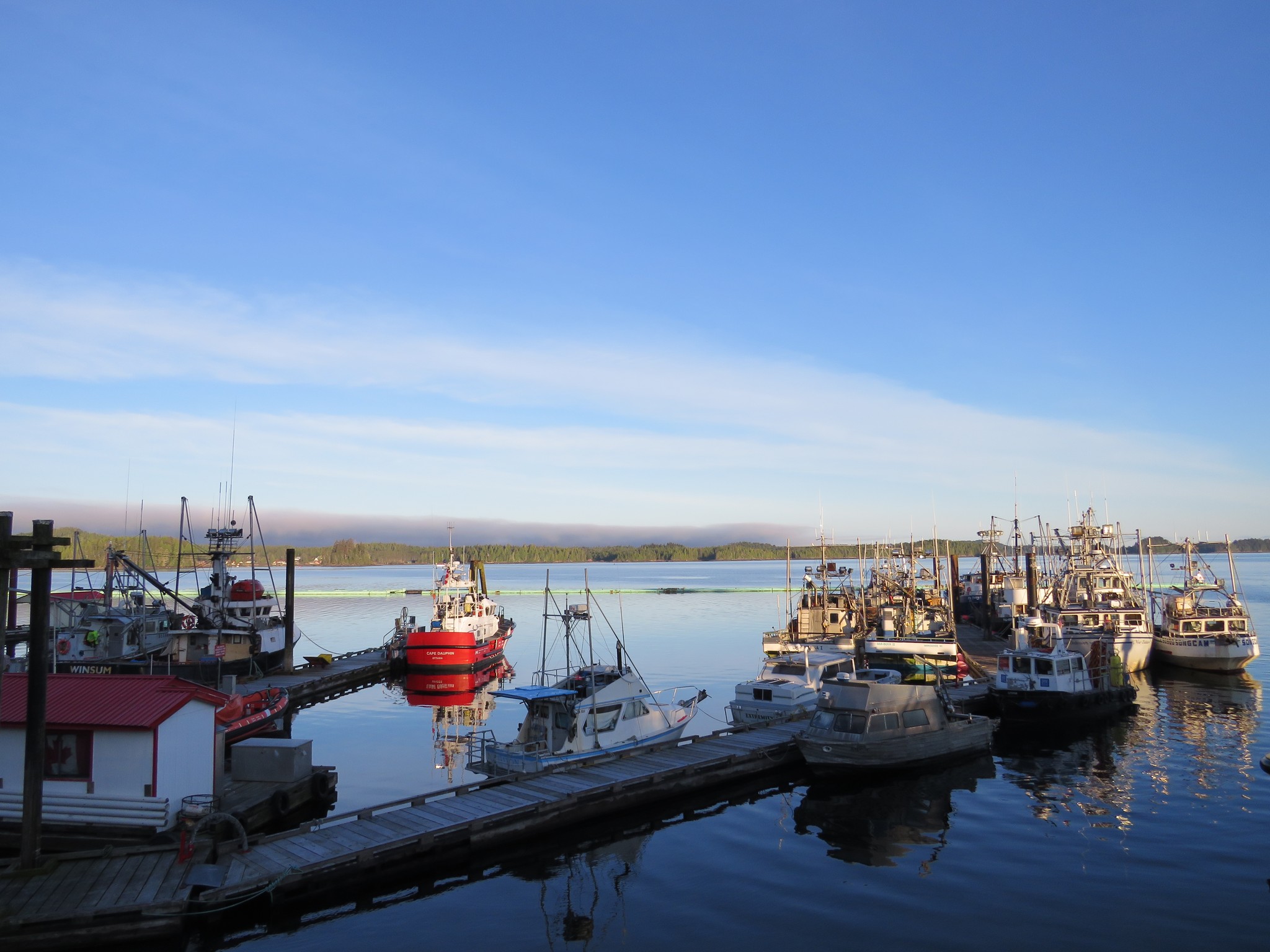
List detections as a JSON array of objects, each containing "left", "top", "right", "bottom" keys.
[
  {"left": 464, "top": 567, "right": 712, "bottom": 782},
  {"left": 388, "top": 522, "right": 517, "bottom": 672},
  {"left": 387, "top": 656, "right": 518, "bottom": 786},
  {"left": 48, "top": 398, "right": 302, "bottom": 681},
  {"left": 723, "top": 476, "right": 1260, "bottom": 782},
  {"left": 213, "top": 686, "right": 289, "bottom": 745}
]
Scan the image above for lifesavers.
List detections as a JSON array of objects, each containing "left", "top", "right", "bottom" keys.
[
  {"left": 182, "top": 614, "right": 195, "bottom": 629},
  {"left": 967, "top": 587, "right": 971, "bottom": 594},
  {"left": 478, "top": 605, "right": 482, "bottom": 617},
  {"left": 56, "top": 638, "right": 72, "bottom": 655},
  {"left": 889, "top": 595, "right": 894, "bottom": 605}
]
